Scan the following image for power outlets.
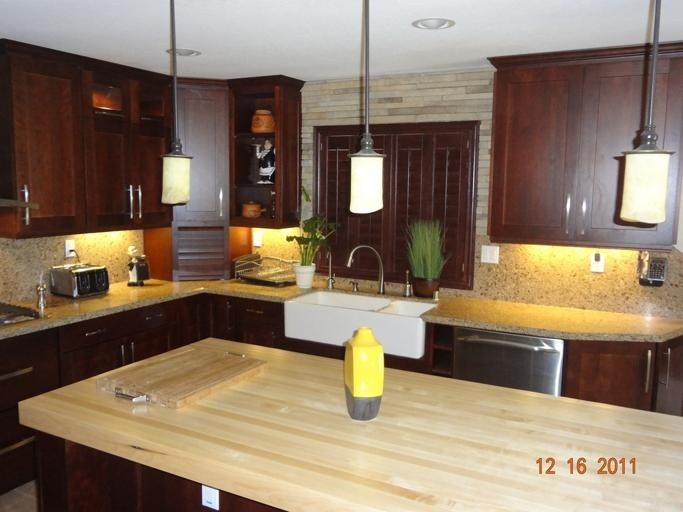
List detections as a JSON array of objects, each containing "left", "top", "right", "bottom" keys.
[{"left": 64, "top": 242, "right": 77, "bottom": 259}]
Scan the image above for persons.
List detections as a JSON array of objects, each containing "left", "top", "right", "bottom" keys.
[{"left": 251, "top": 136, "right": 276, "bottom": 185}]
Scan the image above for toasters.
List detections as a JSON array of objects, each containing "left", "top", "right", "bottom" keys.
[{"left": 48, "top": 263, "right": 109, "bottom": 298}]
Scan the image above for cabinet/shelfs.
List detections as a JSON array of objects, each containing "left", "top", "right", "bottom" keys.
[
  {"left": 88, "top": 55, "right": 174, "bottom": 232},
  {"left": 209, "top": 292, "right": 282, "bottom": 348},
  {"left": 232, "top": 73, "right": 306, "bottom": 230},
  {"left": 16, "top": 335, "right": 681, "bottom": 510},
  {"left": 0, "top": 36, "right": 84, "bottom": 241},
  {"left": 563, "top": 334, "right": 682, "bottom": 419},
  {"left": 60, "top": 294, "right": 204, "bottom": 387},
  {"left": 0, "top": 330, "right": 60, "bottom": 497},
  {"left": 488, "top": 39, "right": 682, "bottom": 252}
]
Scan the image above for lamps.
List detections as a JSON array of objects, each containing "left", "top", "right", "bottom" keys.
[
  {"left": 611, "top": 0, "right": 676, "bottom": 230},
  {"left": 344, "top": 0, "right": 390, "bottom": 216},
  {"left": 154, "top": 3, "right": 193, "bottom": 206}
]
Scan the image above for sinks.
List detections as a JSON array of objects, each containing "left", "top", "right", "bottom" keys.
[
  {"left": 282, "top": 289, "right": 394, "bottom": 354},
  {"left": 377, "top": 295, "right": 437, "bottom": 359}
]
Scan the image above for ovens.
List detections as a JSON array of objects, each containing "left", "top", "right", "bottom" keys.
[{"left": 450, "top": 325, "right": 564, "bottom": 397}]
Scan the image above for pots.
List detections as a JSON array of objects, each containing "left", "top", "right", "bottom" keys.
[{"left": 240, "top": 201, "right": 266, "bottom": 218}]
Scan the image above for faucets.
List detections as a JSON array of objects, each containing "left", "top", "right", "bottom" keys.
[
  {"left": 325, "top": 249, "right": 335, "bottom": 289},
  {"left": 346, "top": 244, "right": 386, "bottom": 295}
]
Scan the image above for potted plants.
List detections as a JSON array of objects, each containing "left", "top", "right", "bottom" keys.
[
  {"left": 286, "top": 214, "right": 338, "bottom": 288},
  {"left": 401, "top": 217, "right": 452, "bottom": 297}
]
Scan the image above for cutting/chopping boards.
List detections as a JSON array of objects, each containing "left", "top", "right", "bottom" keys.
[{"left": 95, "top": 341, "right": 267, "bottom": 411}]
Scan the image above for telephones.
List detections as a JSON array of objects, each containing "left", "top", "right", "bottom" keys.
[{"left": 638, "top": 250, "right": 668, "bottom": 286}]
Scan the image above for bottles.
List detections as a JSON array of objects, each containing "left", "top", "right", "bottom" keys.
[{"left": 34, "top": 285, "right": 47, "bottom": 309}]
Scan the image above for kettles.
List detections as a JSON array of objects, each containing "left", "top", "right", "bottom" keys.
[{"left": 128, "top": 255, "right": 145, "bottom": 286}]
